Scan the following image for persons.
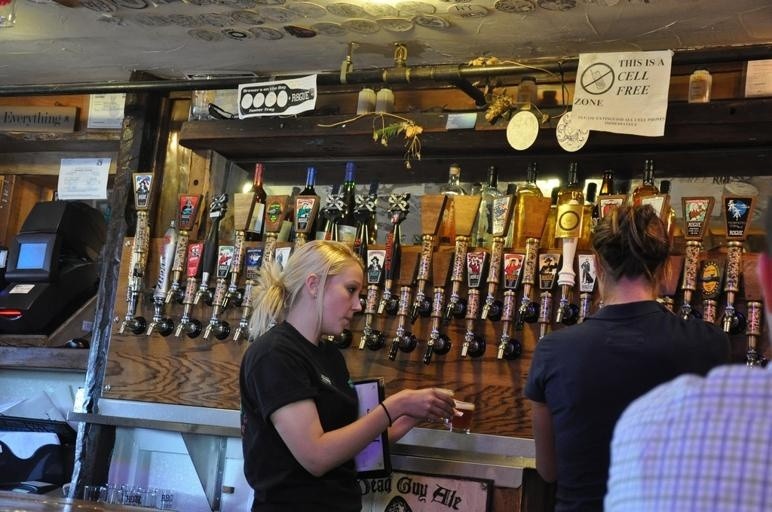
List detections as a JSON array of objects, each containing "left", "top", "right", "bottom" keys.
[
  {"left": 238, "top": 239, "right": 464, "bottom": 512},
  {"left": 523, "top": 203, "right": 736, "bottom": 512},
  {"left": 602, "top": 200, "right": 771, "bottom": 512}
]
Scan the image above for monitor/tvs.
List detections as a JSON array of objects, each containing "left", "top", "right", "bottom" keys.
[{"left": 4, "top": 233, "right": 60, "bottom": 281}]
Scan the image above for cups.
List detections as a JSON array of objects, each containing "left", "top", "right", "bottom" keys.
[
  {"left": 82, "top": 478, "right": 174, "bottom": 511},
  {"left": 190, "top": 88, "right": 215, "bottom": 121},
  {"left": 448, "top": 400, "right": 476, "bottom": 434}
]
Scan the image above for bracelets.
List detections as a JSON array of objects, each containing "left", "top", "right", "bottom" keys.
[{"left": 380, "top": 402, "right": 394, "bottom": 429}]
